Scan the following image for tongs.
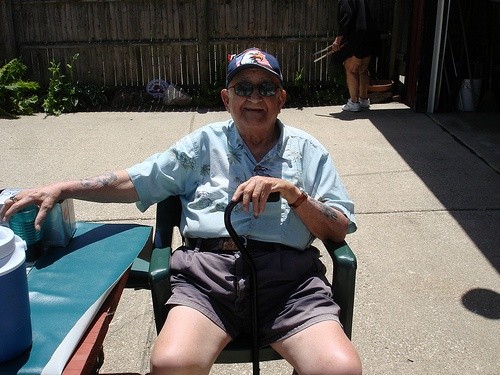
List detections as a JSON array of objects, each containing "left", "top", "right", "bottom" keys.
[{"left": 312, "top": 39, "right": 349, "bottom": 63}]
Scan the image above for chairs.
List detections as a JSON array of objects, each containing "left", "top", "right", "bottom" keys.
[{"left": 148, "top": 195, "right": 357, "bottom": 364}]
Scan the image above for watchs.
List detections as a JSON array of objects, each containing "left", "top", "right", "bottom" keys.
[{"left": 288, "top": 187, "right": 309, "bottom": 209}]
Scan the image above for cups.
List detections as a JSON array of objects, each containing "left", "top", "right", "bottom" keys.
[{"left": 9, "top": 202, "right": 45, "bottom": 262}]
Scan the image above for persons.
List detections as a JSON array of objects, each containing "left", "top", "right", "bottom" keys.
[
  {"left": 0, "top": 48, "right": 363, "bottom": 375},
  {"left": 333, "top": 0, "right": 370, "bottom": 112}
]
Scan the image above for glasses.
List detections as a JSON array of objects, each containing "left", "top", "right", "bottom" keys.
[{"left": 227, "top": 81, "right": 282, "bottom": 96}]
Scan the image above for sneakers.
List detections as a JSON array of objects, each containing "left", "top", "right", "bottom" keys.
[
  {"left": 358, "top": 97, "right": 370, "bottom": 108},
  {"left": 342, "top": 98, "right": 361, "bottom": 112}
]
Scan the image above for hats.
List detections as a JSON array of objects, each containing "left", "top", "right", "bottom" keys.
[{"left": 225, "top": 46, "right": 283, "bottom": 81}]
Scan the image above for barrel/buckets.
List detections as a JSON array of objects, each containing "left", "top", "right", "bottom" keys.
[
  {"left": 454, "top": 76, "right": 483, "bottom": 112},
  {"left": 0, "top": 225, "right": 33, "bottom": 364}
]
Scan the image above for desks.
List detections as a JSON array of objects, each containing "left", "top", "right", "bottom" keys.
[{"left": 0, "top": 222, "right": 154, "bottom": 375}]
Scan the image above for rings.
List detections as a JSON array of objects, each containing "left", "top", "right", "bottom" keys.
[{"left": 10, "top": 196, "right": 18, "bottom": 203}]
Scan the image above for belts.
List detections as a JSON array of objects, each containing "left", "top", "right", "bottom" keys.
[{"left": 185, "top": 236, "right": 293, "bottom": 253}]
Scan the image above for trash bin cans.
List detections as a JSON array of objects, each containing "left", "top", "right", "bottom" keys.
[{"left": 457, "top": 76, "right": 482, "bottom": 111}]
